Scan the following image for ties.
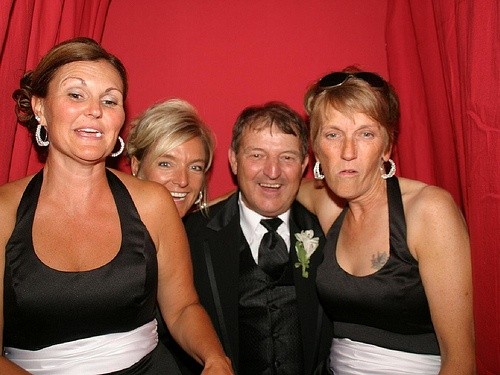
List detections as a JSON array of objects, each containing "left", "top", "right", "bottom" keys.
[{"left": 257, "top": 218, "right": 289, "bottom": 272}]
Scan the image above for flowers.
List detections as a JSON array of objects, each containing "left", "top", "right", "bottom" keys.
[{"left": 294, "top": 230, "right": 319, "bottom": 277}]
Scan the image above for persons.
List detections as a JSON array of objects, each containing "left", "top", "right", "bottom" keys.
[
  {"left": 296, "top": 67, "right": 475, "bottom": 375},
  {"left": 0, "top": 36, "right": 234, "bottom": 375},
  {"left": 180, "top": 102, "right": 334, "bottom": 375},
  {"left": 125, "top": 98, "right": 236, "bottom": 375}
]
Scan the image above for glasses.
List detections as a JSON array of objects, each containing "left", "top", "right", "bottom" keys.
[{"left": 313, "top": 70, "right": 392, "bottom": 103}]
